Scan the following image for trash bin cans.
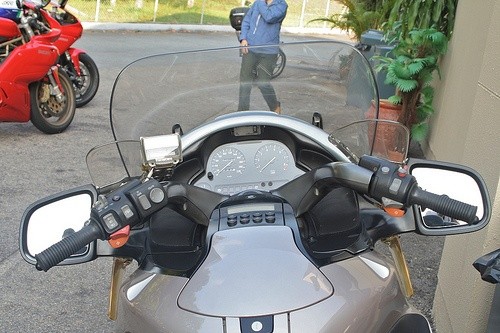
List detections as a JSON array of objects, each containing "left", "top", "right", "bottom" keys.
[{"left": 346, "top": 29, "right": 394, "bottom": 111}]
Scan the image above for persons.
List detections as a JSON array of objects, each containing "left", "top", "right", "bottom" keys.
[
  {"left": 237, "top": 0, "right": 288, "bottom": 114},
  {"left": 60, "top": 227, "right": 86, "bottom": 255},
  {"left": 425, "top": 195, "right": 459, "bottom": 225}
]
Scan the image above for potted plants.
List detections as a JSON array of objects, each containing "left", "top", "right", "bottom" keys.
[{"left": 365, "top": 0, "right": 457, "bottom": 156}]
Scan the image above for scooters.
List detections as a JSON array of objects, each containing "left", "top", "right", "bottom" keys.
[{"left": 229, "top": 7, "right": 285, "bottom": 77}]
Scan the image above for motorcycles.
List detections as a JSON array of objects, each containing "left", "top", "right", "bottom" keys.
[
  {"left": 0, "top": 1, "right": 76, "bottom": 135},
  {"left": 20, "top": 41, "right": 491, "bottom": 333},
  {"left": 0, "top": 0, "right": 100, "bottom": 106}
]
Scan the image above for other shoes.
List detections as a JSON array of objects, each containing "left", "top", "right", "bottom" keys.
[{"left": 274, "top": 102, "right": 281, "bottom": 114}]
